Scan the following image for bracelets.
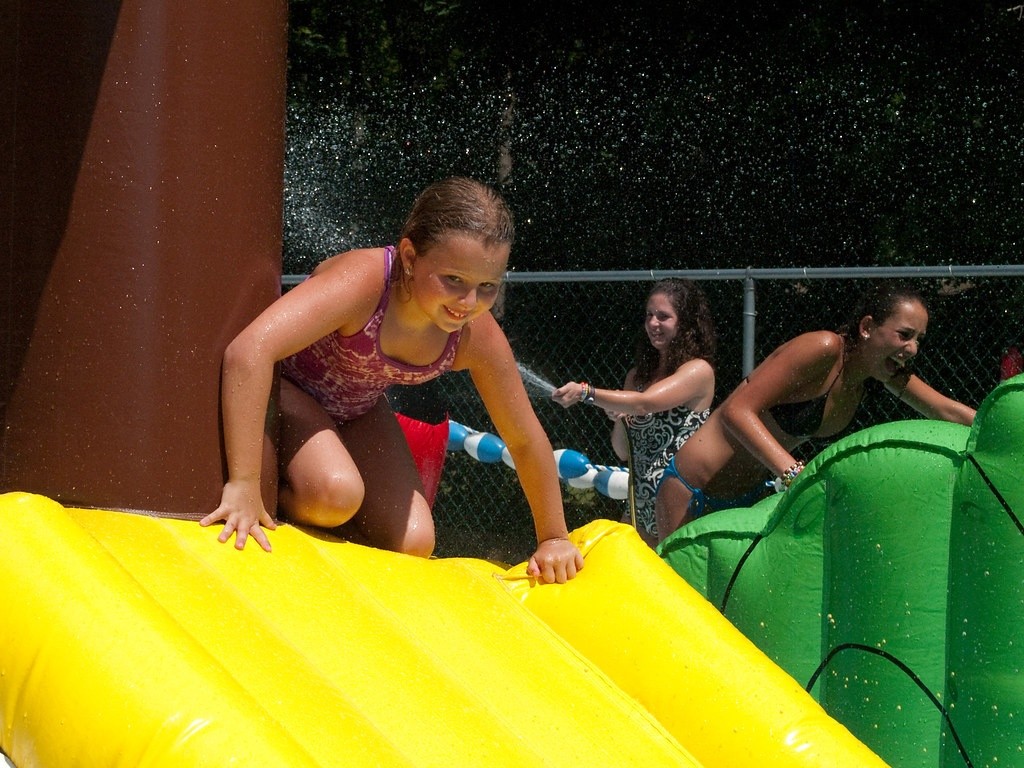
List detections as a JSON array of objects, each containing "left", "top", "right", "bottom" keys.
[
  {"left": 766, "top": 459, "right": 805, "bottom": 493},
  {"left": 581, "top": 383, "right": 595, "bottom": 405}
]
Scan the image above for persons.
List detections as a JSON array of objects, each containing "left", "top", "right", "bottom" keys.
[
  {"left": 655, "top": 284, "right": 977, "bottom": 544},
  {"left": 553, "top": 277, "right": 714, "bottom": 549},
  {"left": 199, "top": 179, "right": 583, "bottom": 584}
]
